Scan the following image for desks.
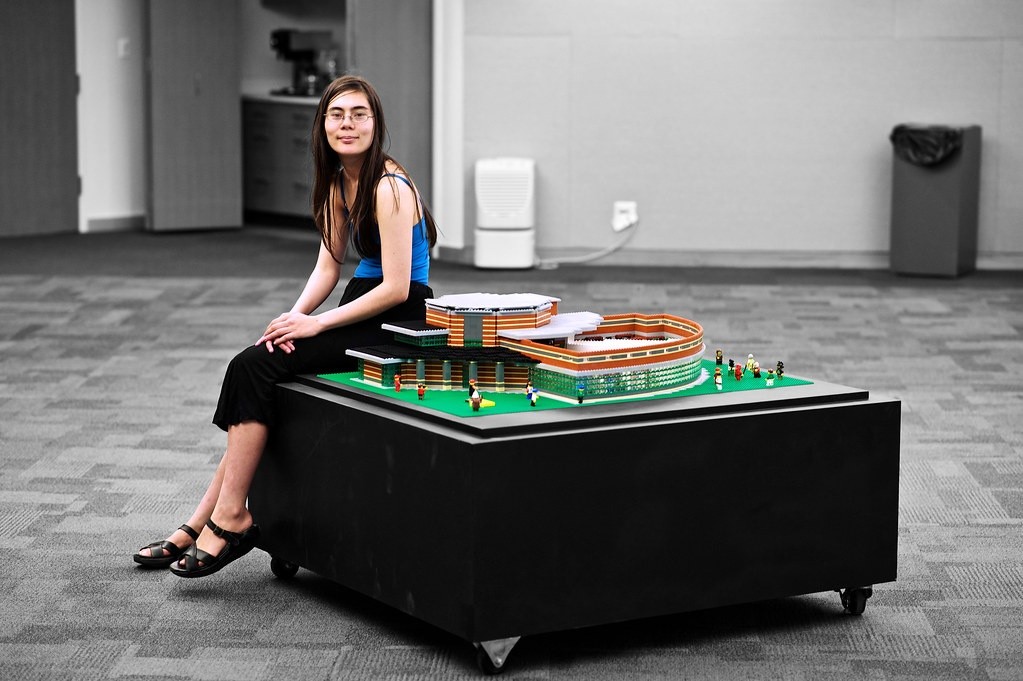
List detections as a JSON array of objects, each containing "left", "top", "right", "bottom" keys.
[{"left": 248, "top": 358, "right": 902, "bottom": 643}]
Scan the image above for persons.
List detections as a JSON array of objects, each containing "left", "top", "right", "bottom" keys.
[
  {"left": 713, "top": 347, "right": 784, "bottom": 390},
  {"left": 134, "top": 73, "right": 437, "bottom": 577},
  {"left": 394, "top": 373, "right": 585, "bottom": 412}
]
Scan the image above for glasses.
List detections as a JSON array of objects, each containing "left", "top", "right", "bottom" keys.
[{"left": 324, "top": 111, "right": 374, "bottom": 123}]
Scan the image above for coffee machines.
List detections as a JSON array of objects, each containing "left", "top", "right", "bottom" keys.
[{"left": 269, "top": 28, "right": 332, "bottom": 96}]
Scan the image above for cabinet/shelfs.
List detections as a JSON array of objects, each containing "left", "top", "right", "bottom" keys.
[{"left": 241, "top": 91, "right": 321, "bottom": 217}]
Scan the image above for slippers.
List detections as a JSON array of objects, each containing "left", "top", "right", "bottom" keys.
[
  {"left": 169, "top": 517, "right": 260, "bottom": 578},
  {"left": 132, "top": 524, "right": 200, "bottom": 565}
]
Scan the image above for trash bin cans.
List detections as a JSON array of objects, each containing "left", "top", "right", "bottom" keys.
[{"left": 888, "top": 122, "right": 984, "bottom": 278}]
